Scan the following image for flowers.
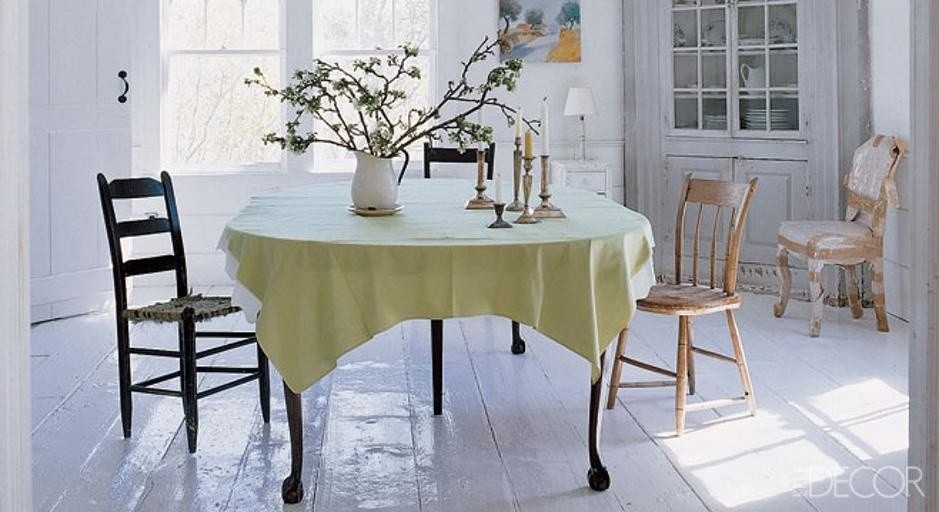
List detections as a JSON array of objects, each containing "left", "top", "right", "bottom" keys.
[{"left": 237, "top": 25, "right": 541, "bottom": 157}]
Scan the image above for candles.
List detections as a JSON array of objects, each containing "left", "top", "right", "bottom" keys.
[
  {"left": 524, "top": 128, "right": 535, "bottom": 156},
  {"left": 474, "top": 100, "right": 489, "bottom": 148},
  {"left": 514, "top": 102, "right": 526, "bottom": 138},
  {"left": 538, "top": 90, "right": 549, "bottom": 156}
]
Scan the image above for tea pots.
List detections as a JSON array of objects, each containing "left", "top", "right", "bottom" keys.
[{"left": 740, "top": 62, "right": 767, "bottom": 96}]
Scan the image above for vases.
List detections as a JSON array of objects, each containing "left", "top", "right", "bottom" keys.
[{"left": 348, "top": 147, "right": 399, "bottom": 216}]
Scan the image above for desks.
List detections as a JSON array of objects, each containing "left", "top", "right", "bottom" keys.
[{"left": 227, "top": 175, "right": 652, "bottom": 505}]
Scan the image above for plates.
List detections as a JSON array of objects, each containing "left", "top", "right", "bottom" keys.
[
  {"left": 757, "top": 17, "right": 791, "bottom": 43},
  {"left": 743, "top": 108, "right": 792, "bottom": 130},
  {"left": 740, "top": 38, "right": 781, "bottom": 45},
  {"left": 703, "top": 20, "right": 725, "bottom": 51},
  {"left": 702, "top": 113, "right": 725, "bottom": 129}
]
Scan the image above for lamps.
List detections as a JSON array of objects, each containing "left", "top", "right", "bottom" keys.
[{"left": 564, "top": 85, "right": 602, "bottom": 162}]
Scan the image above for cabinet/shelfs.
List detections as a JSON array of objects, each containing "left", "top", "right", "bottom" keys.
[
  {"left": 658, "top": 0, "right": 812, "bottom": 133},
  {"left": 550, "top": 159, "right": 609, "bottom": 196},
  {"left": 655, "top": 138, "right": 815, "bottom": 269}
]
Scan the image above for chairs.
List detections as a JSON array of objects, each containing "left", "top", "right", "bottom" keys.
[
  {"left": 768, "top": 132, "right": 904, "bottom": 338},
  {"left": 91, "top": 169, "right": 269, "bottom": 452},
  {"left": 423, "top": 143, "right": 496, "bottom": 180},
  {"left": 604, "top": 169, "right": 760, "bottom": 435}
]
